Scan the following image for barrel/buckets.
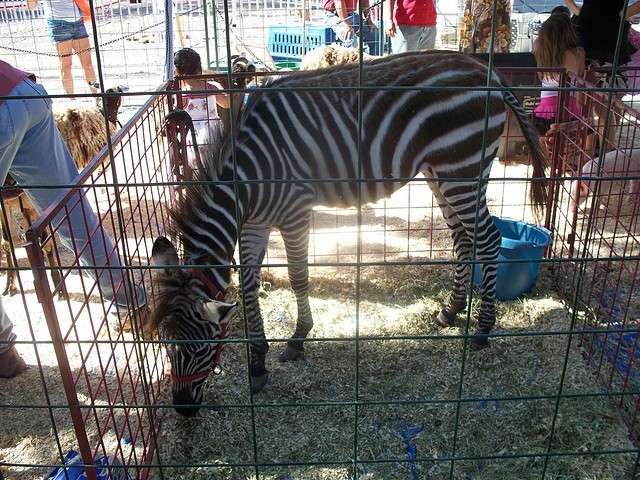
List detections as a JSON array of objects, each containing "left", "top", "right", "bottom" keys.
[{"left": 474, "top": 216, "right": 552, "bottom": 299}]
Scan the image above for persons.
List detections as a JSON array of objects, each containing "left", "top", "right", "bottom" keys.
[
  {"left": 387, "top": 0, "right": 438, "bottom": 52},
  {"left": 535, "top": 14, "right": 595, "bottom": 164},
  {"left": 564, "top": 0, "right": 640, "bottom": 155},
  {"left": 25, "top": 0, "right": 104, "bottom": 110},
  {"left": 174, "top": 48, "right": 239, "bottom": 181},
  {"left": 0, "top": 60, "right": 162, "bottom": 379},
  {"left": 323, "top": 0, "right": 383, "bottom": 57}
]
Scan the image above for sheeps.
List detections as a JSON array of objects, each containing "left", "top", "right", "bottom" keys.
[
  {"left": 299, "top": 43, "right": 382, "bottom": 72},
  {"left": 211, "top": 52, "right": 257, "bottom": 130},
  {"left": 47, "top": 84, "right": 127, "bottom": 166}
]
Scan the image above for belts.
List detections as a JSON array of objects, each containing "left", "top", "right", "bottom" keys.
[{"left": 329, "top": 8, "right": 351, "bottom": 18}]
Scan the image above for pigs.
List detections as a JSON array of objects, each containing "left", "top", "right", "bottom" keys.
[{"left": 571, "top": 150, "right": 640, "bottom": 218}]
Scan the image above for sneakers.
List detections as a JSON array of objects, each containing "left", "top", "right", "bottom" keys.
[
  {"left": 114, "top": 304, "right": 159, "bottom": 341},
  {"left": 0, "top": 346, "right": 26, "bottom": 378}
]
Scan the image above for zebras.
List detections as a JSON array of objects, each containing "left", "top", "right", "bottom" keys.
[{"left": 144, "top": 49, "right": 550, "bottom": 416}]
